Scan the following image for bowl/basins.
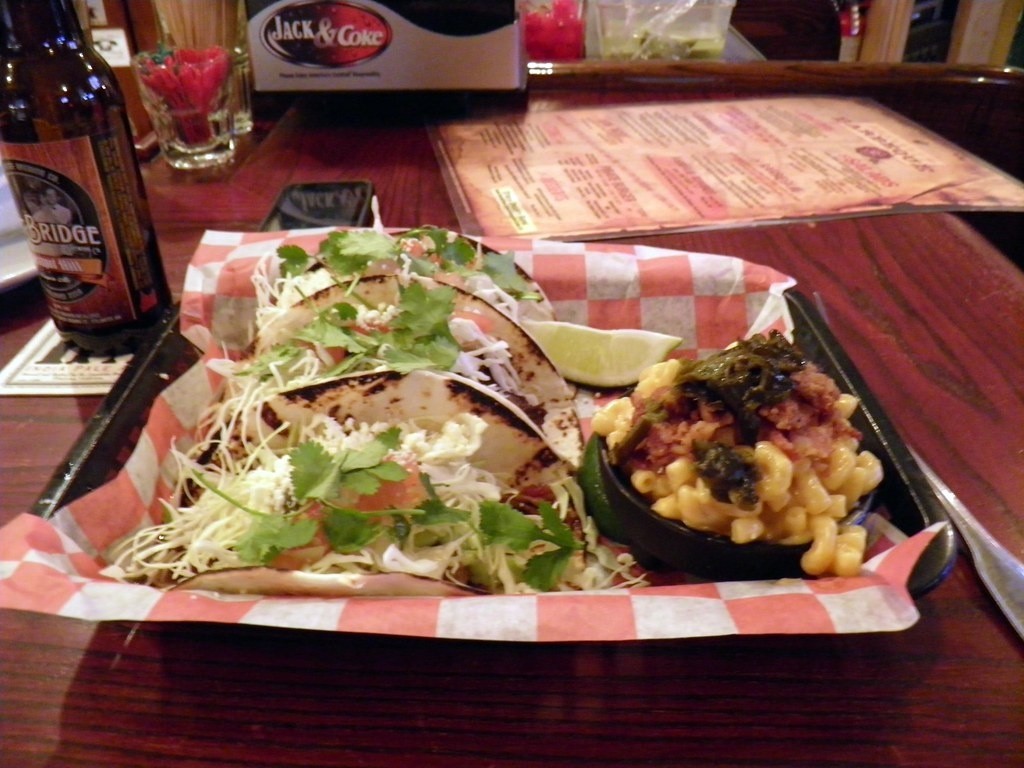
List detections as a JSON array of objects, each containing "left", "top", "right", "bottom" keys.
[
  {"left": 580, "top": 393, "right": 877, "bottom": 583},
  {"left": 593, "top": 0, "right": 736, "bottom": 60}
]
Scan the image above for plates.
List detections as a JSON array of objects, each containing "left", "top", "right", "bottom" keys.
[
  {"left": 255, "top": 181, "right": 374, "bottom": 233},
  {"left": 25, "top": 268, "right": 962, "bottom": 620},
  {"left": 1, "top": 175, "right": 39, "bottom": 289}
]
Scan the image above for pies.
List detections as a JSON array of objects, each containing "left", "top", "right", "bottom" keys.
[{"left": 120, "top": 225, "right": 586, "bottom": 590}]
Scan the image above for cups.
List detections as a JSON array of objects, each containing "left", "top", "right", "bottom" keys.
[
  {"left": 518, "top": 0, "right": 587, "bottom": 64},
  {"left": 130, "top": 47, "right": 236, "bottom": 169},
  {"left": 229, "top": 50, "right": 254, "bottom": 136}
]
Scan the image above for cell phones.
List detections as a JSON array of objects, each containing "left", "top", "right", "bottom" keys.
[{"left": 258, "top": 179, "right": 376, "bottom": 229}]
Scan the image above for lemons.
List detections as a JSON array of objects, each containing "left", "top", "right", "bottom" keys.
[{"left": 520, "top": 316, "right": 683, "bottom": 387}]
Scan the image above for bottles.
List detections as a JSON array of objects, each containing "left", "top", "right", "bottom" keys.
[{"left": 1, "top": 0, "right": 175, "bottom": 358}]
